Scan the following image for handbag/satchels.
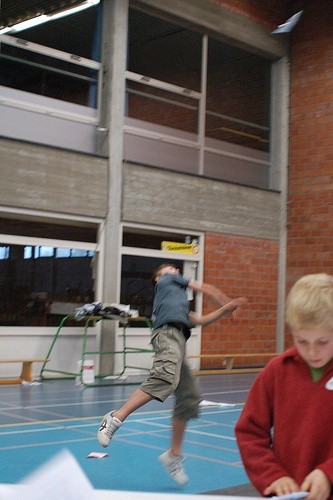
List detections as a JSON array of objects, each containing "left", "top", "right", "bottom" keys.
[{"left": 75, "top": 356, "right": 95, "bottom": 384}]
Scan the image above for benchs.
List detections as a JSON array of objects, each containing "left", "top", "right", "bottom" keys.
[
  {"left": 186, "top": 353, "right": 283, "bottom": 376},
  {"left": 0, "top": 357, "right": 50, "bottom": 385}
]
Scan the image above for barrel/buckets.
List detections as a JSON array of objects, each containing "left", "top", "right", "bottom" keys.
[{"left": 76, "top": 360, "right": 94, "bottom": 385}]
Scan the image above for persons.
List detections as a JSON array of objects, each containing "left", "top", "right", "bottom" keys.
[
  {"left": 235, "top": 274, "right": 333, "bottom": 500},
  {"left": 96, "top": 264, "right": 248, "bottom": 486}
]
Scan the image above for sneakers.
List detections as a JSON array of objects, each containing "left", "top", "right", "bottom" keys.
[
  {"left": 98, "top": 410, "right": 123, "bottom": 448},
  {"left": 158, "top": 448, "right": 189, "bottom": 485}
]
